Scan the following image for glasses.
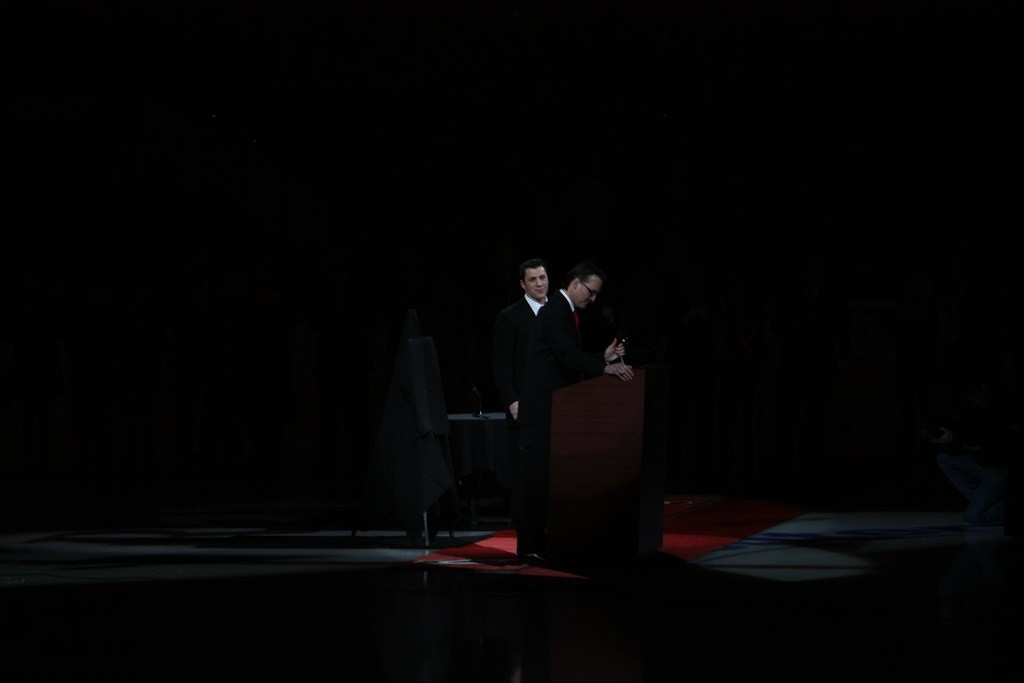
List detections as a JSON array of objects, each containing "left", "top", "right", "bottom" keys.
[{"left": 580, "top": 280, "right": 600, "bottom": 296}]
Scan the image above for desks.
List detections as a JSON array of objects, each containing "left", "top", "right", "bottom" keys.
[{"left": 446, "top": 413, "right": 515, "bottom": 529}]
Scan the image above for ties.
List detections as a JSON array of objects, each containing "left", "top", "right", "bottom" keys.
[{"left": 573, "top": 308, "right": 580, "bottom": 330}]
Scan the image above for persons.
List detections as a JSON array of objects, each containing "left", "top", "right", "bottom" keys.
[{"left": 493, "top": 259, "right": 635, "bottom": 566}]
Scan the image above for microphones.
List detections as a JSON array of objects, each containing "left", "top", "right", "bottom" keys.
[{"left": 600, "top": 305, "right": 622, "bottom": 341}]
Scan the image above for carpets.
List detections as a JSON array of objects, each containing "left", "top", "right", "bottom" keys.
[{"left": 410, "top": 492, "right": 809, "bottom": 579}]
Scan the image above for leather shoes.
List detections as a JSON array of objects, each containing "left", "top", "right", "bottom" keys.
[{"left": 516, "top": 552, "right": 547, "bottom": 567}]
